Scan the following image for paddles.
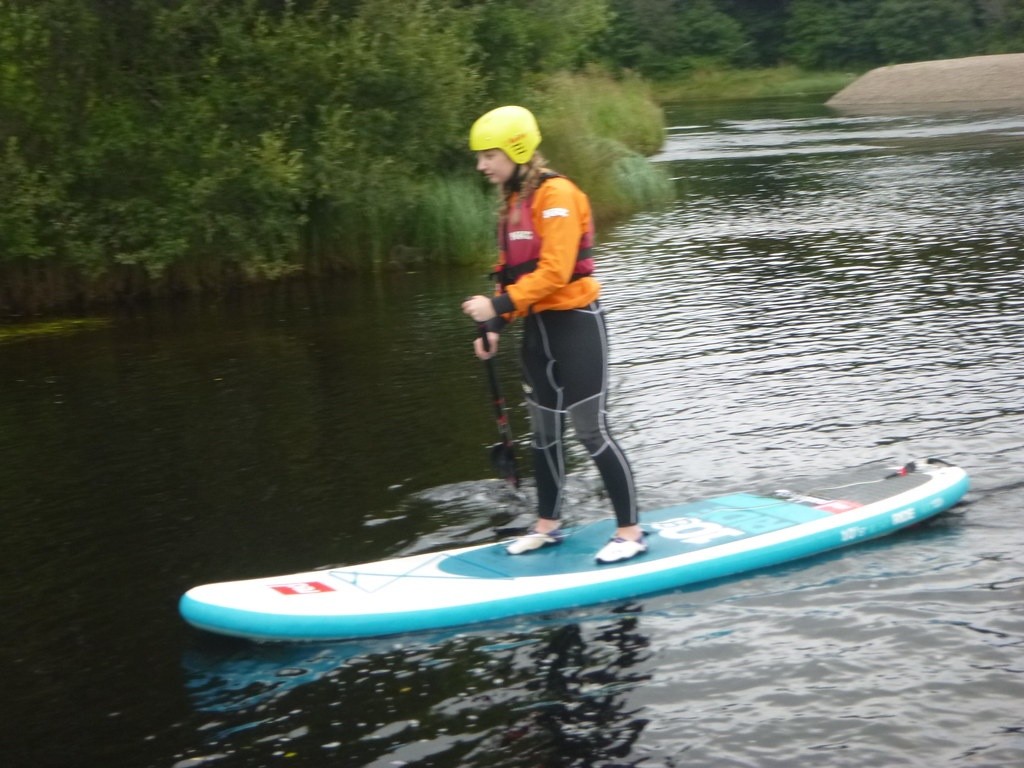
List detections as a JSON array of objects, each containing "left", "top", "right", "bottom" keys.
[{"left": 479, "top": 320, "right": 519, "bottom": 489}]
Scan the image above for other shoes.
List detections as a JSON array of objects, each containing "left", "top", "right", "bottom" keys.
[
  {"left": 595, "top": 529, "right": 646, "bottom": 561},
  {"left": 507, "top": 527, "right": 562, "bottom": 553}
]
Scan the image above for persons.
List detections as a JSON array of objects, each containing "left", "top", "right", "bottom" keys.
[{"left": 462, "top": 105, "right": 649, "bottom": 563}]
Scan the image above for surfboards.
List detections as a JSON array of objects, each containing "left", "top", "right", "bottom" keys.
[{"left": 179, "top": 450, "right": 972, "bottom": 644}]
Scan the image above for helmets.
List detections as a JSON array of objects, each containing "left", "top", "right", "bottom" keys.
[{"left": 469, "top": 105, "right": 542, "bottom": 164}]
